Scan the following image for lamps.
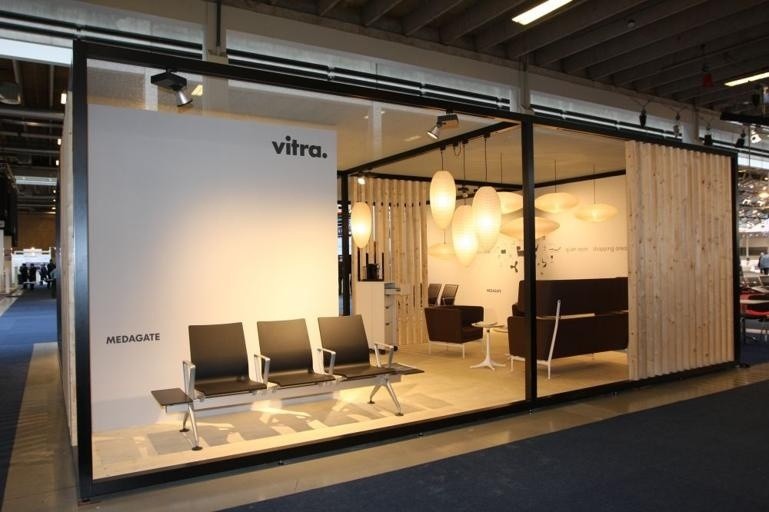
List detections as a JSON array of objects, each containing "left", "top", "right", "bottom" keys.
[
  {"left": 426, "top": 115, "right": 620, "bottom": 265},
  {"left": 149, "top": 68, "right": 193, "bottom": 107},
  {"left": 634, "top": 102, "right": 747, "bottom": 152},
  {"left": 350, "top": 168, "right": 372, "bottom": 250}
]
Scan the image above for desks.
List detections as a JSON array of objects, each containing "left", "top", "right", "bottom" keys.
[
  {"left": 378, "top": 360, "right": 424, "bottom": 380},
  {"left": 149, "top": 387, "right": 193, "bottom": 411},
  {"left": 470, "top": 321, "right": 507, "bottom": 373}
]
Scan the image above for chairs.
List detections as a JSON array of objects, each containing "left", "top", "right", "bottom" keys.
[
  {"left": 413, "top": 283, "right": 423, "bottom": 304},
  {"left": 182, "top": 322, "right": 268, "bottom": 451},
  {"left": 256, "top": 318, "right": 338, "bottom": 402},
  {"left": 426, "top": 283, "right": 442, "bottom": 307},
  {"left": 440, "top": 284, "right": 460, "bottom": 306},
  {"left": 317, "top": 315, "right": 404, "bottom": 417},
  {"left": 424, "top": 305, "right": 486, "bottom": 360}
]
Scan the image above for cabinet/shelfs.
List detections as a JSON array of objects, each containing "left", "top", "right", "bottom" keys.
[{"left": 357, "top": 280, "right": 410, "bottom": 352}]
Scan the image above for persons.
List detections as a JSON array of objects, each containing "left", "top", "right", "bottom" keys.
[
  {"left": 20, "top": 259, "right": 56, "bottom": 291},
  {"left": 758, "top": 249, "right": 769, "bottom": 275}
]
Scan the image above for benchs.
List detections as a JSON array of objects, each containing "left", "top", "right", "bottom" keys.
[{"left": 507, "top": 275, "right": 629, "bottom": 380}]
